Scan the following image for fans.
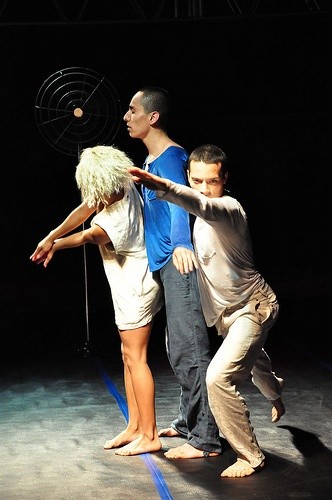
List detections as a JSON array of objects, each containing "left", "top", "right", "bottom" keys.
[{"left": 31, "top": 64, "right": 125, "bottom": 345}]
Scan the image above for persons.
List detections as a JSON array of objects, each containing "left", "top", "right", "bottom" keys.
[
  {"left": 29, "top": 145, "right": 163, "bottom": 457},
  {"left": 127, "top": 146, "right": 287, "bottom": 477},
  {"left": 123, "top": 85, "right": 223, "bottom": 460}
]
